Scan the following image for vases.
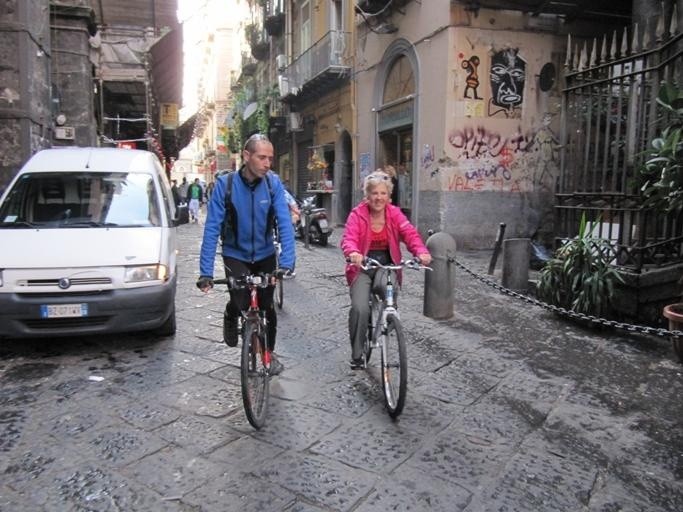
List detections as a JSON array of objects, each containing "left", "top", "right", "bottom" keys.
[{"left": 663, "top": 302, "right": 683, "bottom": 362}]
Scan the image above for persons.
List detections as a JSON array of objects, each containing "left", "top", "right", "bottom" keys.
[
  {"left": 171, "top": 177, "right": 214, "bottom": 223},
  {"left": 276, "top": 188, "right": 299, "bottom": 245},
  {"left": 385, "top": 162, "right": 412, "bottom": 207},
  {"left": 196, "top": 135, "right": 297, "bottom": 376},
  {"left": 340, "top": 173, "right": 431, "bottom": 367}
]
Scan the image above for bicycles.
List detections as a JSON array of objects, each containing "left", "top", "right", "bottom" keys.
[
  {"left": 197, "top": 236, "right": 297, "bottom": 430},
  {"left": 346, "top": 255, "right": 434, "bottom": 418}
]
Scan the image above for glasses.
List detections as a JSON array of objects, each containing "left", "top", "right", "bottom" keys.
[
  {"left": 366, "top": 175, "right": 390, "bottom": 182},
  {"left": 244, "top": 133, "right": 270, "bottom": 150}
]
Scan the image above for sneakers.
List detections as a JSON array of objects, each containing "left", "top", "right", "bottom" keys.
[
  {"left": 223, "top": 310, "right": 239, "bottom": 347},
  {"left": 350, "top": 358, "right": 365, "bottom": 370}
]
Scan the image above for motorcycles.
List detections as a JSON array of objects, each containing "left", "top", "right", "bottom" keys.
[{"left": 293, "top": 195, "right": 333, "bottom": 247}]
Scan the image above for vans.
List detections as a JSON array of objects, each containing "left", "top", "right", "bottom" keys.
[{"left": 0, "top": 147, "right": 189, "bottom": 343}]
